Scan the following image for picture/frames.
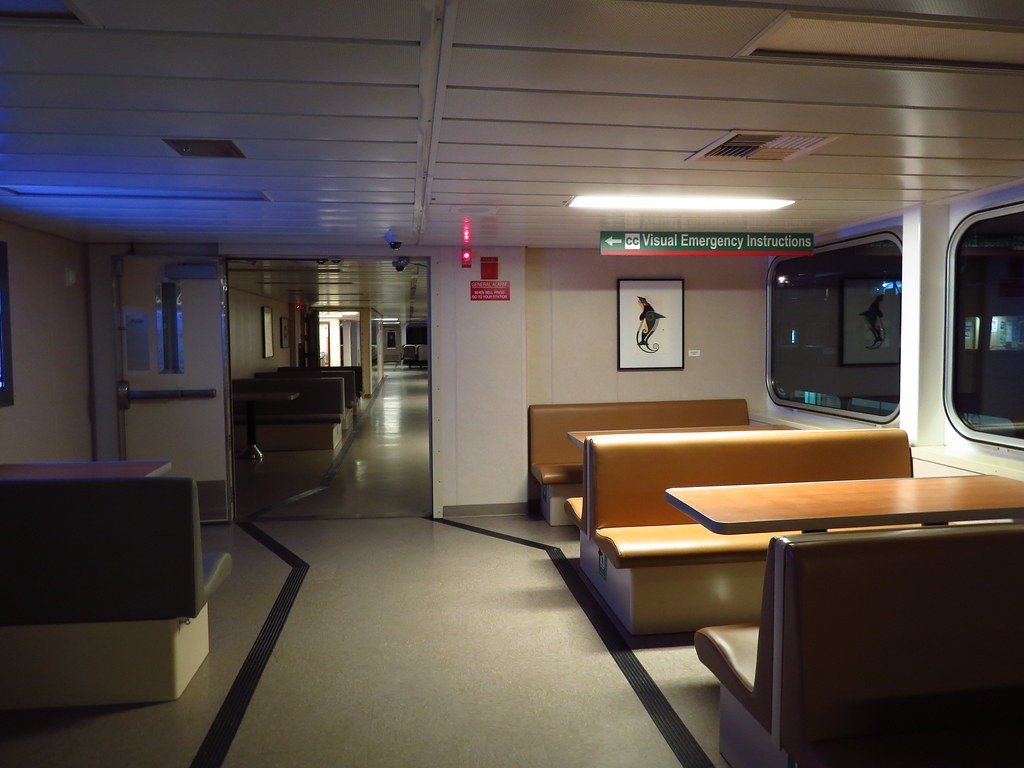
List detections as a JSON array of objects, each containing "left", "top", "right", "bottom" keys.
[
  {"left": 262, "top": 306, "right": 274, "bottom": 358},
  {"left": 280, "top": 317, "right": 290, "bottom": 348},
  {"left": 618, "top": 279, "right": 685, "bottom": 369},
  {"left": 840, "top": 276, "right": 902, "bottom": 367}
]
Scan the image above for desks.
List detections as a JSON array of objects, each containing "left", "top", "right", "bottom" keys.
[
  {"left": 664, "top": 475, "right": 1024, "bottom": 535},
  {"left": 567, "top": 424, "right": 802, "bottom": 450},
  {"left": 0, "top": 461, "right": 171, "bottom": 478},
  {"left": 233, "top": 392, "right": 299, "bottom": 459}
]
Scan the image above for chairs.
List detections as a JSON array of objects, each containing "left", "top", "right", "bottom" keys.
[{"left": 402, "top": 344, "right": 428, "bottom": 369}]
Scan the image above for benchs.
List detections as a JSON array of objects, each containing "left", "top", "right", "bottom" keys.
[
  {"left": 779, "top": 528, "right": 1024, "bottom": 768},
  {"left": 692, "top": 534, "right": 884, "bottom": 768},
  {"left": 233, "top": 366, "right": 365, "bottom": 451},
  {"left": 563, "top": 435, "right": 590, "bottom": 582},
  {"left": 0, "top": 477, "right": 233, "bottom": 710},
  {"left": 590, "top": 429, "right": 955, "bottom": 631},
  {"left": 528, "top": 398, "right": 748, "bottom": 528}
]
